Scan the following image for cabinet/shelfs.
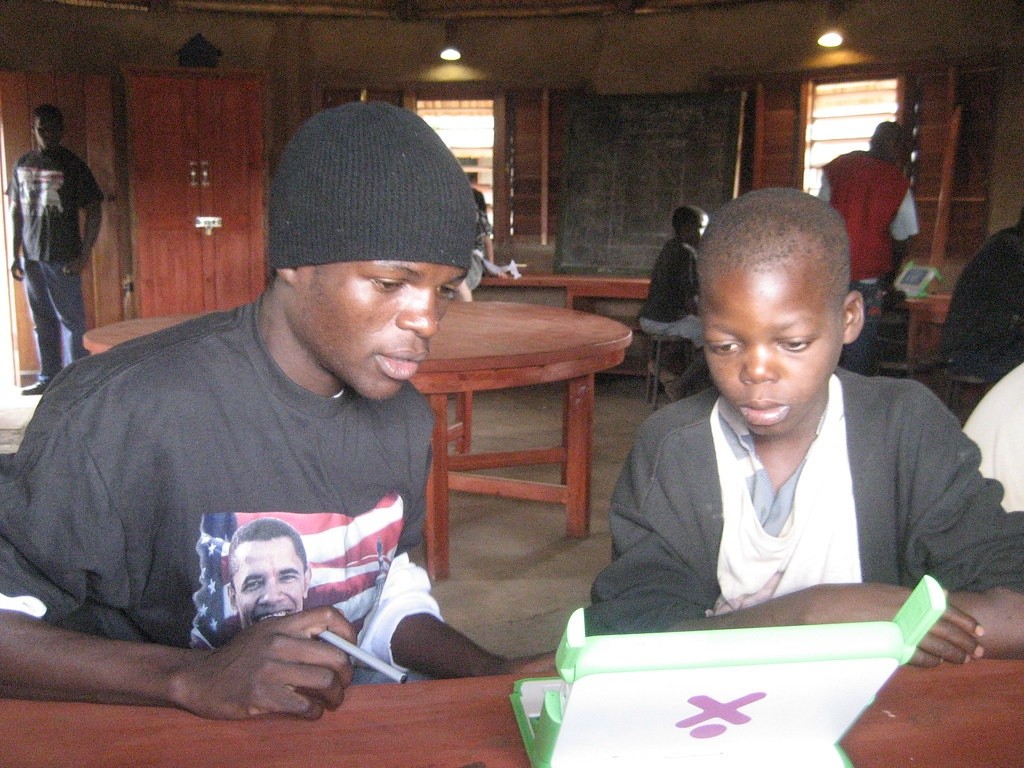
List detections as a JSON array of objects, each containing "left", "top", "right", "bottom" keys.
[{"left": 123, "top": 63, "right": 269, "bottom": 319}]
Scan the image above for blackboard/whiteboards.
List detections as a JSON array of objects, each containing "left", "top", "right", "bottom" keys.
[{"left": 552, "top": 89, "right": 746, "bottom": 276}]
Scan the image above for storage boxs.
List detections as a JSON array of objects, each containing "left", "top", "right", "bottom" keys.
[{"left": 509, "top": 572, "right": 947, "bottom": 768}]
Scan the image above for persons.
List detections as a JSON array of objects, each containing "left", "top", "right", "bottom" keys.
[
  {"left": 0, "top": 101, "right": 558, "bottom": 718},
  {"left": 437, "top": 121, "right": 1024, "bottom": 403},
  {"left": 587, "top": 189, "right": 1024, "bottom": 669},
  {"left": 4, "top": 104, "right": 104, "bottom": 395}
]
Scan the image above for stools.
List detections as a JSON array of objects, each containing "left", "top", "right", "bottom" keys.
[
  {"left": 645, "top": 336, "right": 691, "bottom": 410},
  {"left": 946, "top": 375, "right": 982, "bottom": 408}
]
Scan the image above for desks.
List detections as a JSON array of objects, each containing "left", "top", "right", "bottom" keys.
[
  {"left": 82, "top": 301, "right": 632, "bottom": 578},
  {"left": 0, "top": 657, "right": 1024, "bottom": 768}
]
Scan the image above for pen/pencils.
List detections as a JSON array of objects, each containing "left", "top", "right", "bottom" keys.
[{"left": 320, "top": 630, "right": 408, "bottom": 683}]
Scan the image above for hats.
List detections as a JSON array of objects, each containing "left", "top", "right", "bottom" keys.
[{"left": 269, "top": 101, "right": 476, "bottom": 269}]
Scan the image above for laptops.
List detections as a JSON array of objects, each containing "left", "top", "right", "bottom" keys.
[
  {"left": 509, "top": 575, "right": 947, "bottom": 768},
  {"left": 893, "top": 261, "right": 942, "bottom": 300}
]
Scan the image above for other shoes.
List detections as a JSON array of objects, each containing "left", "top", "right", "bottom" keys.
[{"left": 20, "top": 381, "right": 49, "bottom": 395}]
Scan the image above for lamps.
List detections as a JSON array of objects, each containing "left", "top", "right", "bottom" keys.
[
  {"left": 815, "top": 5, "right": 845, "bottom": 46},
  {"left": 440, "top": 20, "right": 461, "bottom": 61}
]
